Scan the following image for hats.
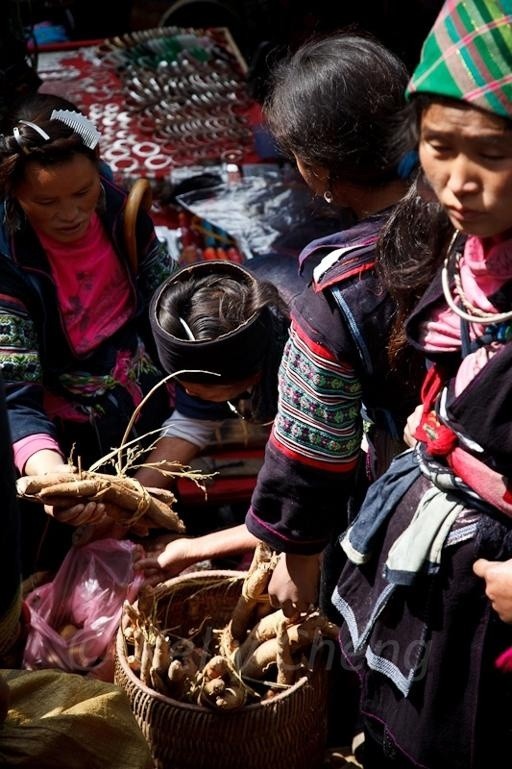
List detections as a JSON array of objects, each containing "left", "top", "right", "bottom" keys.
[
  {"left": 146, "top": 255, "right": 269, "bottom": 387},
  {"left": 401, "top": 0, "right": 512, "bottom": 125}
]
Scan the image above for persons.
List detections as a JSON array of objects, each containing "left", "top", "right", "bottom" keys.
[
  {"left": 334, "top": 0, "right": 510, "bottom": 769},
  {"left": 65, "top": 245, "right": 304, "bottom": 578},
  {"left": 3, "top": 92, "right": 179, "bottom": 544},
  {"left": 232, "top": 26, "right": 425, "bottom": 618}
]
